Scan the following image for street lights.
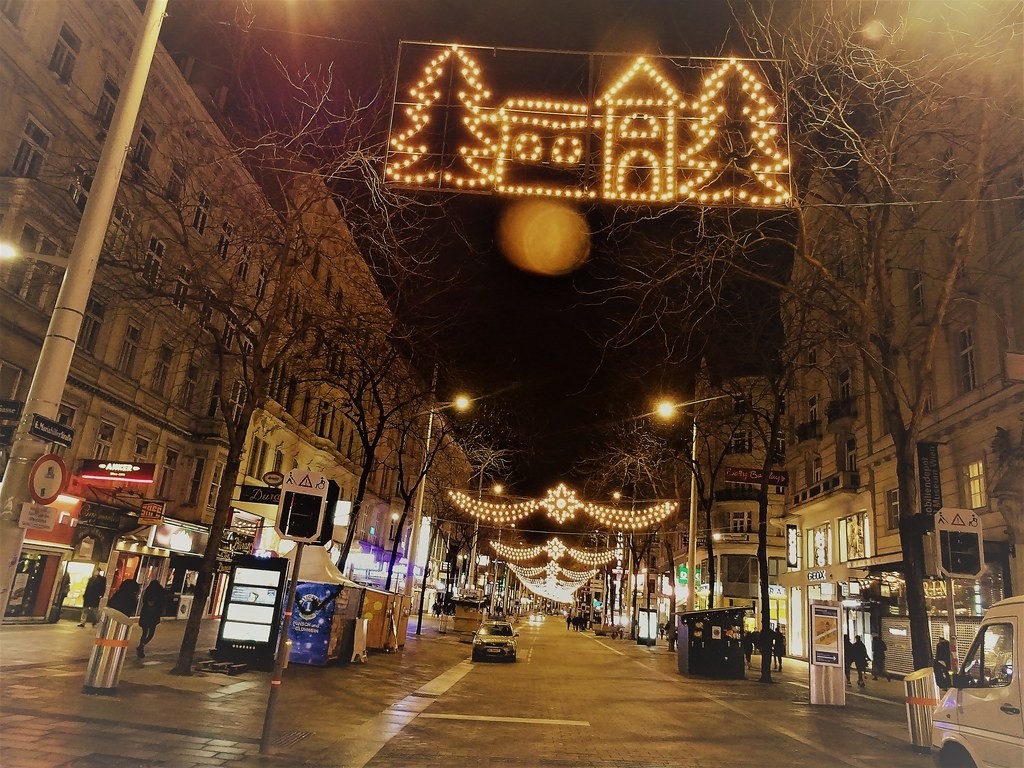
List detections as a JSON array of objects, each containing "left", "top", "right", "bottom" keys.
[
  {"left": 613, "top": 491, "right": 636, "bottom": 635},
  {"left": 654, "top": 402, "right": 699, "bottom": 613},
  {"left": 469, "top": 485, "right": 501, "bottom": 591},
  {"left": 503, "top": 541, "right": 523, "bottom": 616},
  {"left": 398, "top": 396, "right": 472, "bottom": 597},
  {"left": 490, "top": 523, "right": 516, "bottom": 616},
  {"left": 594, "top": 530, "right": 609, "bottom": 628}
]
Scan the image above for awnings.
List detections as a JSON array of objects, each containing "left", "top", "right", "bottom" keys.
[{"left": 847, "top": 551, "right": 903, "bottom": 577}]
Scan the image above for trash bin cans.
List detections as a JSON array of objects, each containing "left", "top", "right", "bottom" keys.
[
  {"left": 82, "top": 608, "right": 134, "bottom": 693},
  {"left": 902, "top": 667, "right": 942, "bottom": 753}
]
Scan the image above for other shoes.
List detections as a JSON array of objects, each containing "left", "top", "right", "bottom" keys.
[
  {"left": 92, "top": 623, "right": 98, "bottom": 628},
  {"left": 857, "top": 680, "right": 860, "bottom": 685},
  {"left": 846, "top": 682, "right": 852, "bottom": 687},
  {"left": 872, "top": 678, "right": 878, "bottom": 681},
  {"left": 774, "top": 667, "right": 778, "bottom": 669},
  {"left": 887, "top": 676, "right": 892, "bottom": 682},
  {"left": 748, "top": 666, "right": 750, "bottom": 669},
  {"left": 137, "top": 645, "right": 146, "bottom": 658},
  {"left": 775, "top": 670, "right": 783, "bottom": 672},
  {"left": 860, "top": 682, "right": 865, "bottom": 688},
  {"left": 78, "top": 624, "right": 84, "bottom": 627}
]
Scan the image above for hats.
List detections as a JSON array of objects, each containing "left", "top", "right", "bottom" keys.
[
  {"left": 97, "top": 567, "right": 102, "bottom": 570},
  {"left": 855, "top": 635, "right": 860, "bottom": 639},
  {"left": 844, "top": 635, "right": 850, "bottom": 640},
  {"left": 145, "top": 580, "right": 163, "bottom": 591}
]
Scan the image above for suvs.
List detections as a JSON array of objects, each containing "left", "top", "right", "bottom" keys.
[{"left": 471, "top": 619, "right": 519, "bottom": 664}]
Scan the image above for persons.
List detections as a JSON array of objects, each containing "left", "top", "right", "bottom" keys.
[
  {"left": 432, "top": 602, "right": 442, "bottom": 618},
  {"left": 107, "top": 579, "right": 165, "bottom": 659},
  {"left": 844, "top": 632, "right": 891, "bottom": 686},
  {"left": 659, "top": 621, "right": 670, "bottom": 640},
  {"left": 935, "top": 635, "right": 950, "bottom": 670},
  {"left": 58, "top": 567, "right": 106, "bottom": 627},
  {"left": 566, "top": 615, "right": 587, "bottom": 632},
  {"left": 675, "top": 627, "right": 678, "bottom": 646},
  {"left": 744, "top": 627, "right": 786, "bottom": 672}
]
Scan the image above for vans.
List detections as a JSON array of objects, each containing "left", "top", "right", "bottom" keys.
[{"left": 928, "top": 593, "right": 1024, "bottom": 768}]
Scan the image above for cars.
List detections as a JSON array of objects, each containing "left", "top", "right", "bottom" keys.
[{"left": 530, "top": 611, "right": 546, "bottom": 622}]
